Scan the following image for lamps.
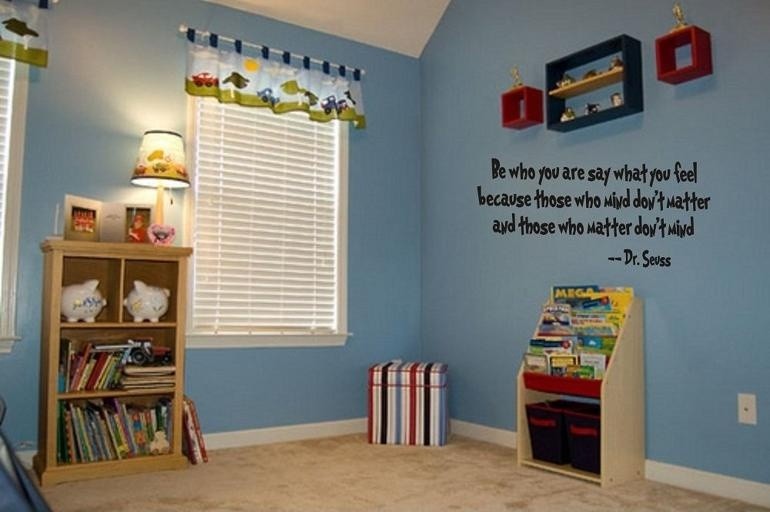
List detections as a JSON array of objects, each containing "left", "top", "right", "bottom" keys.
[{"left": 131, "top": 130, "right": 191, "bottom": 225}]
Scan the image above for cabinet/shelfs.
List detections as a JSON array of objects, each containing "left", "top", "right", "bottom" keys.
[
  {"left": 517, "top": 295, "right": 646, "bottom": 488},
  {"left": 544, "top": 33, "right": 643, "bottom": 134},
  {"left": 32, "top": 241, "right": 193, "bottom": 489}
]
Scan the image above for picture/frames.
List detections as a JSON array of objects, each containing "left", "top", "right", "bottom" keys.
[
  {"left": 124, "top": 204, "right": 154, "bottom": 244},
  {"left": 148, "top": 225, "right": 176, "bottom": 245},
  {"left": 63, "top": 194, "right": 104, "bottom": 241}
]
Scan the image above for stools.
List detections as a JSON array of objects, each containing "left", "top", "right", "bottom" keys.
[{"left": 367, "top": 360, "right": 449, "bottom": 447}]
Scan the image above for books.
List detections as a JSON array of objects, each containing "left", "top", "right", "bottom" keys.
[
  {"left": 56, "top": 397, "right": 173, "bottom": 465},
  {"left": 523, "top": 286, "right": 629, "bottom": 377},
  {"left": 58, "top": 337, "right": 130, "bottom": 393}
]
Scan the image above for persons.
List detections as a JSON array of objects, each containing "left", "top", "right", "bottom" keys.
[{"left": 127, "top": 215, "right": 146, "bottom": 244}]
